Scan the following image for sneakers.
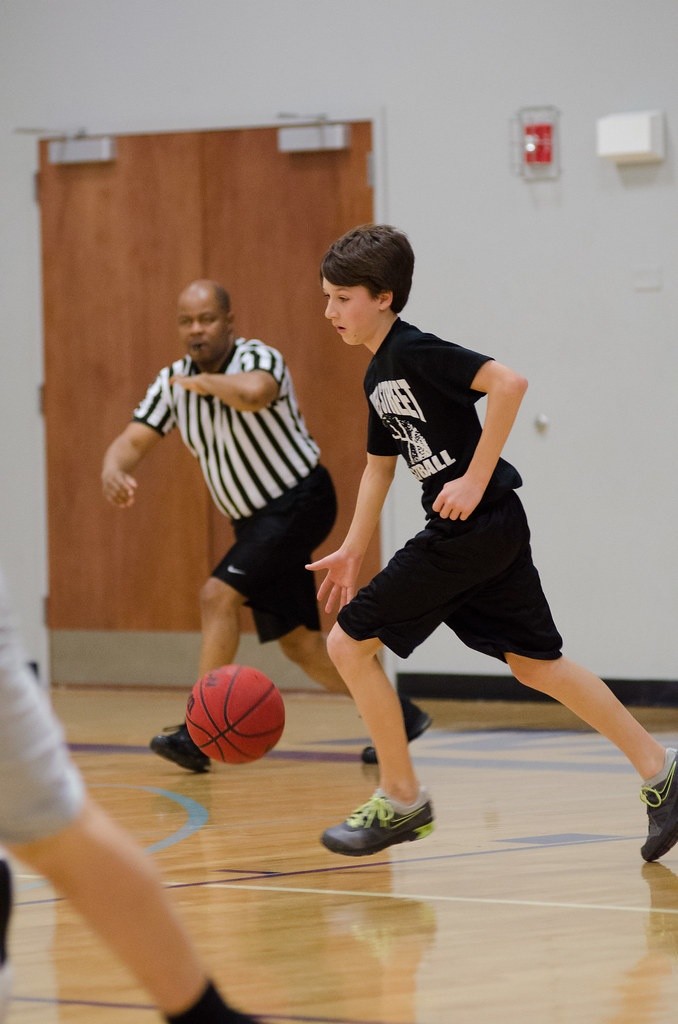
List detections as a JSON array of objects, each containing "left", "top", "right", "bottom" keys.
[
  {"left": 320, "top": 786, "right": 434, "bottom": 856},
  {"left": 361, "top": 695, "right": 433, "bottom": 764},
  {"left": 151, "top": 721, "right": 211, "bottom": 772},
  {"left": 640, "top": 748, "right": 678, "bottom": 862}
]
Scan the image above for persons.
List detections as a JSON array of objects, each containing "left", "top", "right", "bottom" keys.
[
  {"left": 102, "top": 279, "right": 432, "bottom": 772},
  {"left": 0, "top": 612, "right": 264, "bottom": 1024},
  {"left": 305, "top": 224, "right": 678, "bottom": 861}
]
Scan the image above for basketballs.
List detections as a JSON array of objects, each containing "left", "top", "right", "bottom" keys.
[{"left": 185, "top": 663, "right": 287, "bottom": 764}]
